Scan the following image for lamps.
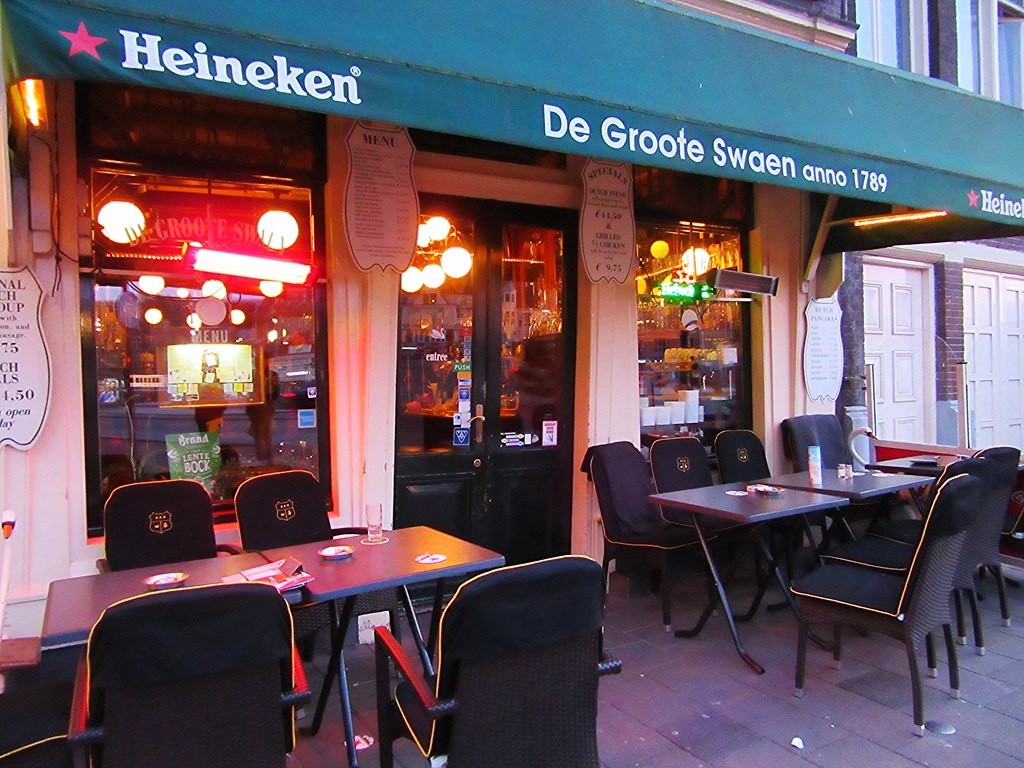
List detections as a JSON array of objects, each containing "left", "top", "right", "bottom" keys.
[
  {"left": 682, "top": 309, "right": 698, "bottom": 331},
  {"left": 6, "top": 76, "right": 49, "bottom": 152}
]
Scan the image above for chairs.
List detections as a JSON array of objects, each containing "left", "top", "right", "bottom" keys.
[
  {"left": 65, "top": 582, "right": 312, "bottom": 768},
  {"left": 579, "top": 414, "right": 895, "bottom": 633},
  {"left": 865, "top": 446, "right": 1022, "bottom": 645},
  {"left": 96, "top": 469, "right": 404, "bottom": 681},
  {"left": 818, "top": 458, "right": 993, "bottom": 676},
  {"left": 374, "top": 554, "right": 622, "bottom": 768},
  {"left": 790, "top": 474, "right": 982, "bottom": 738}
]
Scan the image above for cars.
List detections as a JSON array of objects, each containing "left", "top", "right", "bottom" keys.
[{"left": 268, "top": 352, "right": 316, "bottom": 409}]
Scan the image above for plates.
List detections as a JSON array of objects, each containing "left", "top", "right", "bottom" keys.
[
  {"left": 755, "top": 487, "right": 786, "bottom": 494},
  {"left": 317, "top": 545, "right": 356, "bottom": 560},
  {"left": 639, "top": 389, "right": 699, "bottom": 426},
  {"left": 142, "top": 572, "right": 189, "bottom": 590}
]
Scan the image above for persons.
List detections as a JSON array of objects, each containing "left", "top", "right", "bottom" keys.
[{"left": 195, "top": 350, "right": 280, "bottom": 465}]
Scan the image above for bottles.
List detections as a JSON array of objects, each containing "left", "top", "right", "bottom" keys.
[
  {"left": 837, "top": 464, "right": 845, "bottom": 478},
  {"left": 845, "top": 465, "right": 853, "bottom": 479}
]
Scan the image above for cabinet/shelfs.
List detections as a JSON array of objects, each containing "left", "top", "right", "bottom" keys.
[{"left": 646, "top": 365, "right": 739, "bottom": 421}]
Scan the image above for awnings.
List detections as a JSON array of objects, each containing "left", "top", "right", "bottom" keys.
[{"left": 2, "top": 1, "right": 1023, "bottom": 258}]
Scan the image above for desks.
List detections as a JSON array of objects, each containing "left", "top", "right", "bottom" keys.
[
  {"left": 748, "top": 469, "right": 937, "bottom": 637},
  {"left": 262, "top": 525, "right": 505, "bottom": 768},
  {"left": 866, "top": 454, "right": 1023, "bottom": 520},
  {"left": 647, "top": 482, "right": 850, "bottom": 673},
  {"left": 37, "top": 552, "right": 301, "bottom": 651}
]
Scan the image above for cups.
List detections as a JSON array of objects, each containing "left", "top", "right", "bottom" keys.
[{"left": 366, "top": 504, "right": 383, "bottom": 541}]
